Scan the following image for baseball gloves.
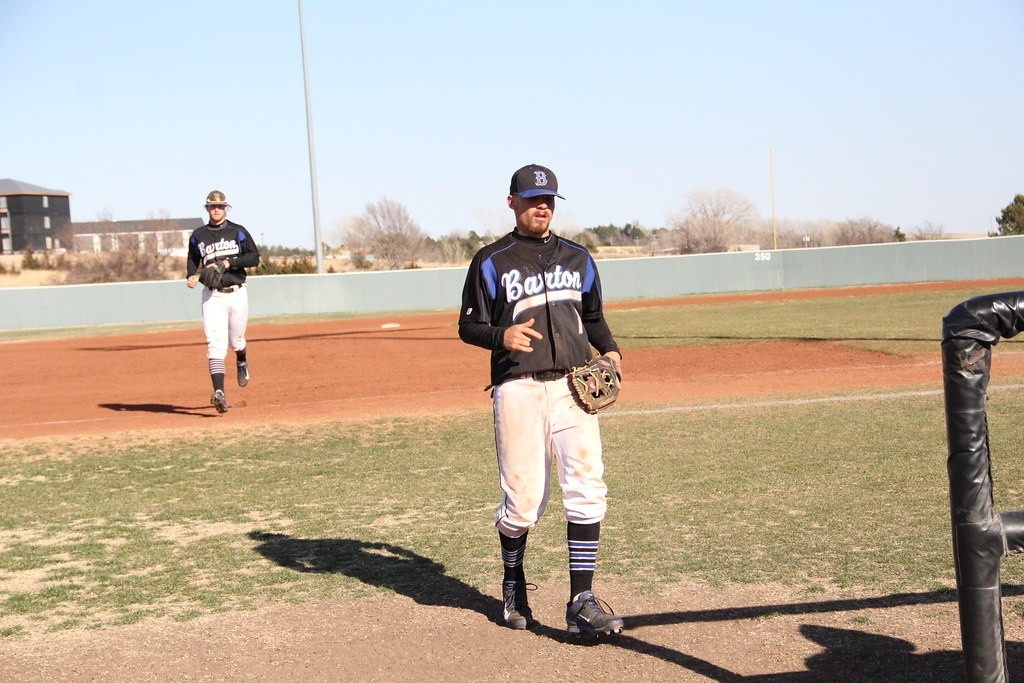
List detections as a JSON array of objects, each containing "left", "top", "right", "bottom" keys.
[
  {"left": 198, "top": 259, "right": 226, "bottom": 291},
  {"left": 565, "top": 357, "right": 622, "bottom": 414}
]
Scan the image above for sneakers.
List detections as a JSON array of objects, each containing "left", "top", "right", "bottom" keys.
[
  {"left": 237, "top": 362, "right": 249, "bottom": 387},
  {"left": 211, "top": 392, "right": 227, "bottom": 413},
  {"left": 503, "top": 574, "right": 538, "bottom": 630},
  {"left": 565, "top": 590, "right": 624, "bottom": 638}
]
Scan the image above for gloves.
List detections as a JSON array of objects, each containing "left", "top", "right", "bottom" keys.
[{"left": 201, "top": 258, "right": 226, "bottom": 288}]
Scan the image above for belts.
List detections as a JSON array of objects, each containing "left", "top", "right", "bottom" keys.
[
  {"left": 208, "top": 283, "right": 242, "bottom": 294},
  {"left": 511, "top": 369, "right": 576, "bottom": 382}
]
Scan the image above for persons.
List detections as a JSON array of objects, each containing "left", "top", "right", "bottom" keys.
[
  {"left": 184, "top": 190, "right": 261, "bottom": 414},
  {"left": 459, "top": 164, "right": 629, "bottom": 640}
]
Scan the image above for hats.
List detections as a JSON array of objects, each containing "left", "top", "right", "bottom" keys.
[
  {"left": 509, "top": 164, "right": 566, "bottom": 199},
  {"left": 205, "top": 190, "right": 228, "bottom": 206}
]
